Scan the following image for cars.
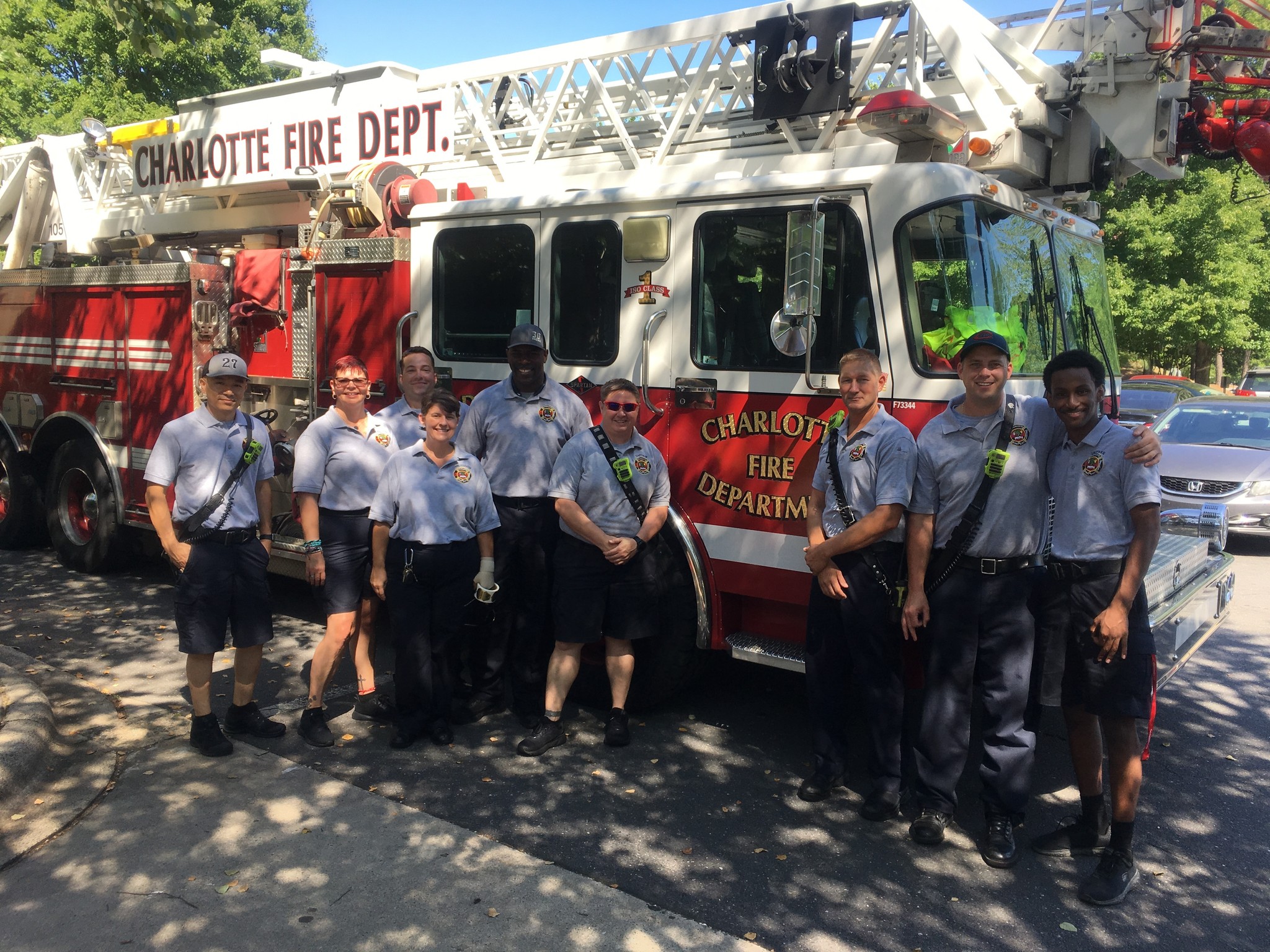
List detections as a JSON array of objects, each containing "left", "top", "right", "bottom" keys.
[
  {"left": 1144, "top": 391, "right": 1270, "bottom": 537},
  {"left": 1118, "top": 367, "right": 1269, "bottom": 433}
]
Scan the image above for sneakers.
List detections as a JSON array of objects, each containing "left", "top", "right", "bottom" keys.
[
  {"left": 223, "top": 701, "right": 286, "bottom": 739},
  {"left": 351, "top": 695, "right": 397, "bottom": 725},
  {"left": 296, "top": 706, "right": 335, "bottom": 748},
  {"left": 188, "top": 714, "right": 234, "bottom": 757},
  {"left": 516, "top": 716, "right": 567, "bottom": 757},
  {"left": 1077, "top": 847, "right": 1141, "bottom": 906},
  {"left": 602, "top": 705, "right": 631, "bottom": 747},
  {"left": 1033, "top": 815, "right": 1112, "bottom": 858}
]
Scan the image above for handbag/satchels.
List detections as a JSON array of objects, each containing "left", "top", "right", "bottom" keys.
[{"left": 158, "top": 411, "right": 252, "bottom": 572}]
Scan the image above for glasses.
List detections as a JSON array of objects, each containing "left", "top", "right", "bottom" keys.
[
  {"left": 603, "top": 401, "right": 641, "bottom": 412},
  {"left": 335, "top": 377, "right": 369, "bottom": 387}
]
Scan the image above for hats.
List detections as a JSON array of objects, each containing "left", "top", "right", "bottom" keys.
[
  {"left": 952, "top": 330, "right": 1011, "bottom": 367},
  {"left": 507, "top": 323, "right": 546, "bottom": 352},
  {"left": 201, "top": 353, "right": 251, "bottom": 382}
]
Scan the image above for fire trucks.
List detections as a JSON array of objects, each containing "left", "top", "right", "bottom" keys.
[{"left": 0, "top": 0, "right": 1268, "bottom": 714}]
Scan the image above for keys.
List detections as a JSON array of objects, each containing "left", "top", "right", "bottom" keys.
[{"left": 403, "top": 563, "right": 418, "bottom": 583}]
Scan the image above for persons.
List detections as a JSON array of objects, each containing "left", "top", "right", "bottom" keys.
[
  {"left": 518, "top": 378, "right": 672, "bottom": 757},
  {"left": 141, "top": 353, "right": 286, "bottom": 757},
  {"left": 901, "top": 329, "right": 1163, "bottom": 871},
  {"left": 292, "top": 354, "right": 402, "bottom": 747},
  {"left": 455, "top": 324, "right": 593, "bottom": 730},
  {"left": 373, "top": 345, "right": 470, "bottom": 449},
  {"left": 797, "top": 348, "right": 919, "bottom": 822},
  {"left": 368, "top": 391, "right": 501, "bottom": 751},
  {"left": 1029, "top": 348, "right": 1162, "bottom": 906}
]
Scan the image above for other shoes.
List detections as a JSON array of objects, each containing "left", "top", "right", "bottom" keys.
[
  {"left": 391, "top": 721, "right": 425, "bottom": 749},
  {"left": 457, "top": 696, "right": 505, "bottom": 721},
  {"left": 513, "top": 702, "right": 542, "bottom": 727},
  {"left": 426, "top": 719, "right": 454, "bottom": 745}
]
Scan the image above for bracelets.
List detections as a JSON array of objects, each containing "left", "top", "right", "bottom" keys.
[{"left": 301, "top": 539, "right": 322, "bottom": 560}]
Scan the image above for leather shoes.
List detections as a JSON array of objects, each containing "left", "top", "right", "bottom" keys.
[
  {"left": 857, "top": 784, "right": 912, "bottom": 821},
  {"left": 908, "top": 808, "right": 954, "bottom": 845},
  {"left": 981, "top": 813, "right": 1020, "bottom": 869},
  {"left": 795, "top": 771, "right": 848, "bottom": 802}
]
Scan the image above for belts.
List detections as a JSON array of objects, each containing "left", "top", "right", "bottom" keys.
[
  {"left": 181, "top": 524, "right": 257, "bottom": 545},
  {"left": 957, "top": 553, "right": 1045, "bottom": 574},
  {"left": 492, "top": 494, "right": 554, "bottom": 510},
  {"left": 1047, "top": 558, "right": 1127, "bottom": 581}
]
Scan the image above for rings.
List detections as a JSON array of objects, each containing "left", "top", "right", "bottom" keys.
[
  {"left": 309, "top": 574, "right": 315, "bottom": 578},
  {"left": 178, "top": 567, "right": 181, "bottom": 570}
]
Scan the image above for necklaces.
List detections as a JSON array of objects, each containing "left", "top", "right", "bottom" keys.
[{"left": 335, "top": 406, "right": 368, "bottom": 430}]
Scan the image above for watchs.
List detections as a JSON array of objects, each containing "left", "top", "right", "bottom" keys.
[
  {"left": 632, "top": 535, "right": 647, "bottom": 552},
  {"left": 260, "top": 534, "right": 275, "bottom": 543}
]
